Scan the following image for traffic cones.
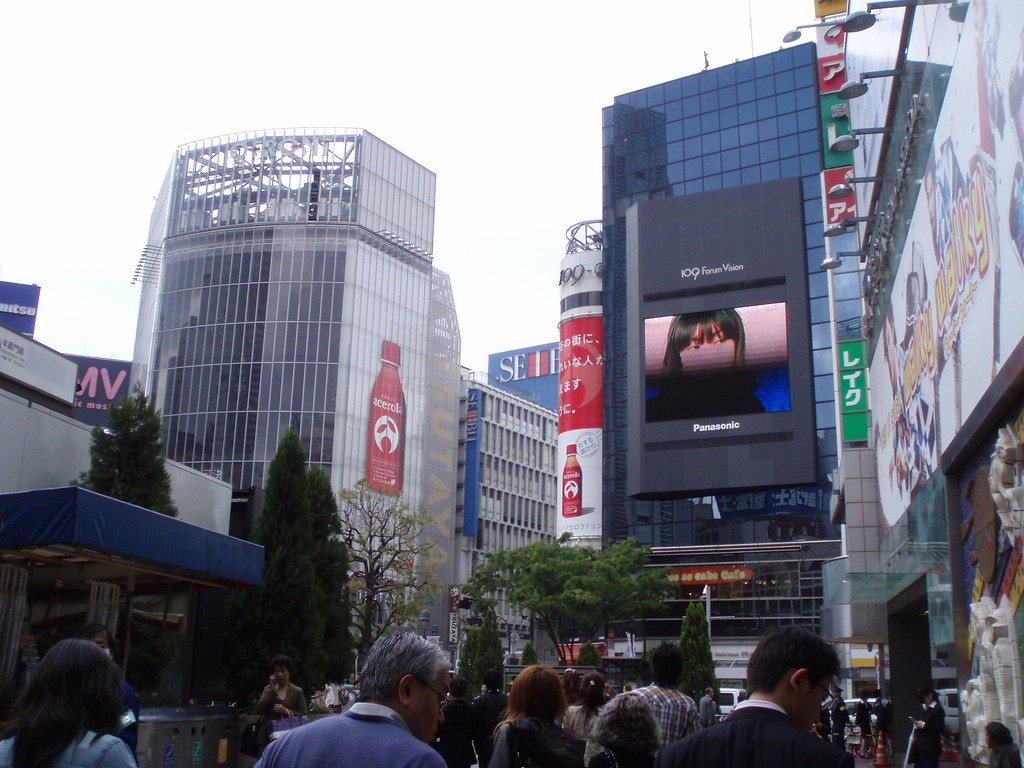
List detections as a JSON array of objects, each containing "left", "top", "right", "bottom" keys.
[
  {"left": 874, "top": 729, "right": 890, "bottom": 768},
  {"left": 937, "top": 725, "right": 961, "bottom": 762},
  {"left": 841, "top": 728, "right": 873, "bottom": 768}
]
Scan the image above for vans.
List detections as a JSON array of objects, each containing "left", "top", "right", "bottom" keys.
[
  {"left": 718, "top": 688, "right": 748, "bottom": 723},
  {"left": 933, "top": 688, "right": 960, "bottom": 736},
  {"left": 823, "top": 697, "right": 878, "bottom": 728}
]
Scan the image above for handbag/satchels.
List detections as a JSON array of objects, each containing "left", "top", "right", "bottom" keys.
[
  {"left": 264, "top": 708, "right": 309, "bottom": 742},
  {"left": 240, "top": 706, "right": 281, "bottom": 757}
]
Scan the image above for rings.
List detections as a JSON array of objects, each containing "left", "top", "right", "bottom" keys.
[{"left": 277, "top": 706, "right": 278, "bottom": 708}]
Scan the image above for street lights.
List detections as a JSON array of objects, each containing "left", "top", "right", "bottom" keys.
[
  {"left": 455, "top": 596, "right": 568, "bottom": 666},
  {"left": 418, "top": 605, "right": 431, "bottom": 639}
]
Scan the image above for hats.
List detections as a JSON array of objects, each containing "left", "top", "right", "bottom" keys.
[{"left": 830, "top": 681, "right": 844, "bottom": 694}]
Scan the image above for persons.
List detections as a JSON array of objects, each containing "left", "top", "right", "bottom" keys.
[
  {"left": 910, "top": 687, "right": 944, "bottom": 768},
  {"left": 253, "top": 633, "right": 450, "bottom": 768},
  {"left": 855, "top": 690, "right": 875, "bottom": 760},
  {"left": 0, "top": 622, "right": 138, "bottom": 768},
  {"left": 986, "top": 721, "right": 1022, "bottom": 768},
  {"left": 255, "top": 657, "right": 307, "bottom": 740},
  {"left": 436, "top": 625, "right": 855, "bottom": 768},
  {"left": 662, "top": 307, "right": 746, "bottom": 372}
]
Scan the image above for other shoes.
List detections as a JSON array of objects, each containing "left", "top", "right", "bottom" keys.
[{"left": 860, "top": 754, "right": 869, "bottom": 759}]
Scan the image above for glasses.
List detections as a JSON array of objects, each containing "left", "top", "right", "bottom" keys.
[
  {"left": 811, "top": 677, "right": 834, "bottom": 711},
  {"left": 397, "top": 673, "right": 452, "bottom": 709}
]
